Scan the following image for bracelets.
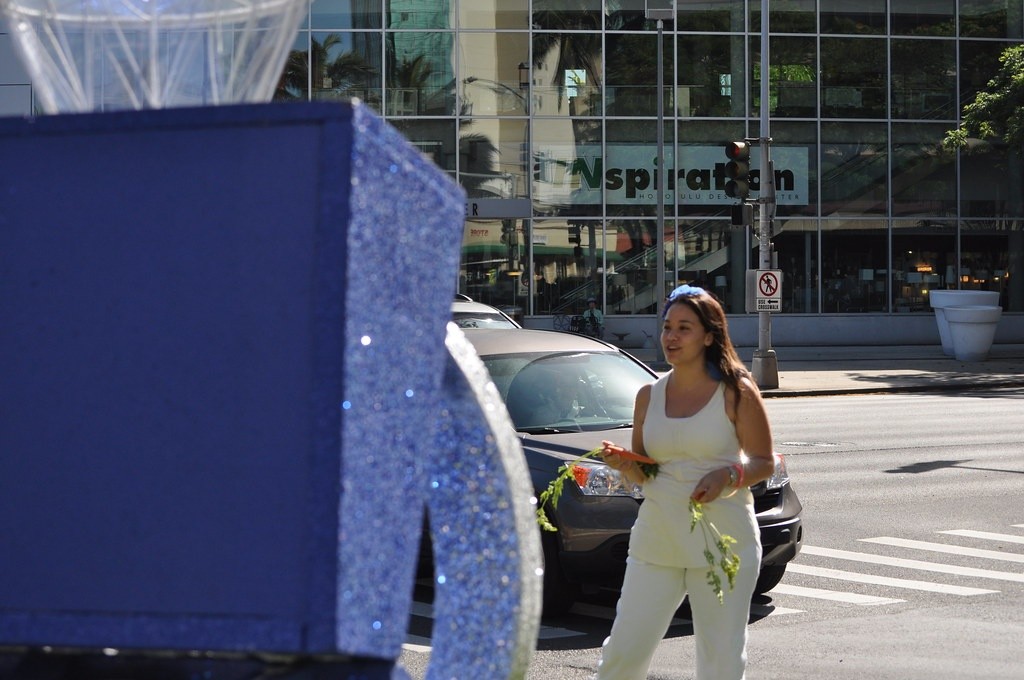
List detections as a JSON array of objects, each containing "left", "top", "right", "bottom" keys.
[{"left": 731, "top": 464, "right": 744, "bottom": 487}]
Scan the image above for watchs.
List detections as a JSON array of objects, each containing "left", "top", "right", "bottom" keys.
[{"left": 726, "top": 466, "right": 737, "bottom": 486}]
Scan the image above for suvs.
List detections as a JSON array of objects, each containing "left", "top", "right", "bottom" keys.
[{"left": 450, "top": 291, "right": 521, "bottom": 332}]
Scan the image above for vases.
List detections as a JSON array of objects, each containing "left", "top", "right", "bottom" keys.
[{"left": 943, "top": 304, "right": 1003, "bottom": 361}]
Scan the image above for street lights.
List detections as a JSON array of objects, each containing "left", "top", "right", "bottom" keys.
[{"left": 466, "top": 77, "right": 532, "bottom": 277}]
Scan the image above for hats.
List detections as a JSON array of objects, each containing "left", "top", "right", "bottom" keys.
[{"left": 587, "top": 297, "right": 596, "bottom": 302}]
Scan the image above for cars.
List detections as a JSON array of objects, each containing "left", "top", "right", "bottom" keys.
[{"left": 419, "top": 326, "right": 804, "bottom": 610}]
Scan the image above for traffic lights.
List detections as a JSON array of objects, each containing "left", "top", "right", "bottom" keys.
[
  {"left": 566, "top": 219, "right": 582, "bottom": 244},
  {"left": 574, "top": 246, "right": 584, "bottom": 257},
  {"left": 723, "top": 141, "right": 750, "bottom": 199},
  {"left": 732, "top": 203, "right": 753, "bottom": 226}
]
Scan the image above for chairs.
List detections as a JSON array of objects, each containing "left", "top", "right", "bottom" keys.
[
  {"left": 368, "top": 87, "right": 416, "bottom": 116},
  {"left": 552, "top": 315, "right": 601, "bottom": 339}
]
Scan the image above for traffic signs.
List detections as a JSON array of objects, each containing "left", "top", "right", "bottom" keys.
[{"left": 754, "top": 268, "right": 782, "bottom": 312}]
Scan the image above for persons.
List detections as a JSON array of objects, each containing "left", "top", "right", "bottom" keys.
[
  {"left": 528, "top": 371, "right": 597, "bottom": 426},
  {"left": 584, "top": 298, "right": 605, "bottom": 340},
  {"left": 595, "top": 284, "right": 774, "bottom": 680}
]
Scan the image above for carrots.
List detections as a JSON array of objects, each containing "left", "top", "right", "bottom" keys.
[{"left": 604, "top": 445, "right": 656, "bottom": 464}]
